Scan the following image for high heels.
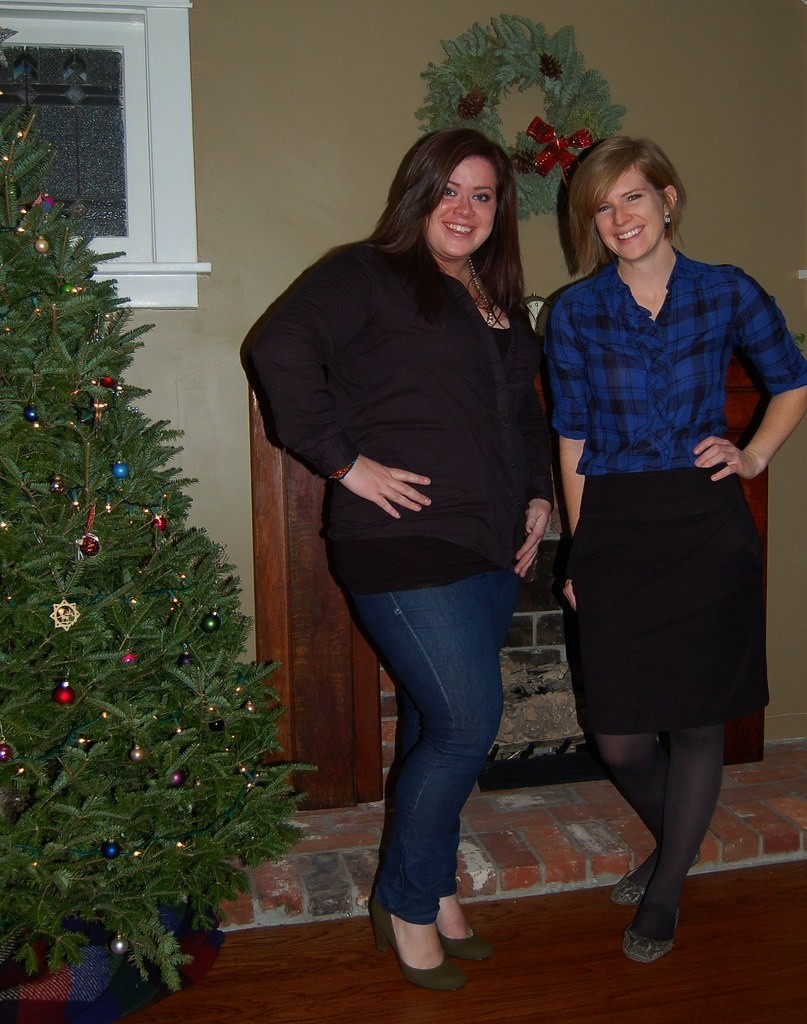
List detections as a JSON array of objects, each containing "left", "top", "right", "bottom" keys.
[{"left": 367, "top": 890, "right": 492, "bottom": 991}]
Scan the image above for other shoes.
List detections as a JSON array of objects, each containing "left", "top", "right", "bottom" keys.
[
  {"left": 620, "top": 905, "right": 680, "bottom": 964},
  {"left": 609, "top": 846, "right": 702, "bottom": 906}
]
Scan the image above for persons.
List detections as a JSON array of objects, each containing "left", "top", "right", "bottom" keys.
[
  {"left": 545, "top": 134, "right": 807, "bottom": 964},
  {"left": 240, "top": 128, "right": 556, "bottom": 991}
]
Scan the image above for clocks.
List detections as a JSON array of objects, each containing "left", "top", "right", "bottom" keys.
[{"left": 524, "top": 293, "right": 546, "bottom": 332}]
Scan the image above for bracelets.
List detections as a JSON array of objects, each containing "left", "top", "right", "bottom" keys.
[{"left": 329, "top": 460, "right": 355, "bottom": 482}]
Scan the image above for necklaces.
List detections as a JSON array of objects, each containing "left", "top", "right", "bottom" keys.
[{"left": 467, "top": 255, "right": 493, "bottom": 324}]
